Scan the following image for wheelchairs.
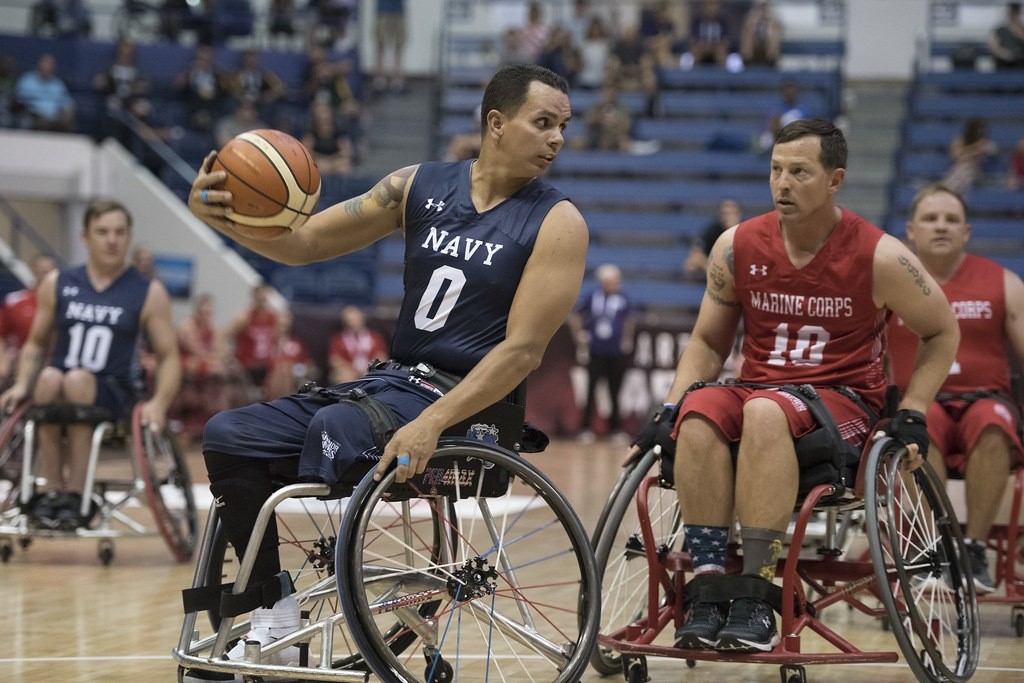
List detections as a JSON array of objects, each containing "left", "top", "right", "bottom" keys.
[
  {"left": 172, "top": 419, "right": 602, "bottom": 683},
  {"left": 827, "top": 434, "right": 1024, "bottom": 636},
  {"left": 570, "top": 401, "right": 984, "bottom": 683},
  {"left": 0, "top": 401, "right": 202, "bottom": 563}
]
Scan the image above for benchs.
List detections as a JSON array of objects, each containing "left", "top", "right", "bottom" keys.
[{"left": 0, "top": 32, "right": 1024, "bottom": 311}]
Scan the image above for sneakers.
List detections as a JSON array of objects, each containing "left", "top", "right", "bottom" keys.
[
  {"left": 912, "top": 548, "right": 953, "bottom": 594},
  {"left": 954, "top": 541, "right": 996, "bottom": 596},
  {"left": 183, "top": 596, "right": 317, "bottom": 683},
  {"left": 673, "top": 597, "right": 734, "bottom": 648},
  {"left": 715, "top": 598, "right": 782, "bottom": 652}
]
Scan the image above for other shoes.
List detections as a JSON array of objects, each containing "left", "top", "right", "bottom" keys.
[
  {"left": 53, "top": 489, "right": 82, "bottom": 531},
  {"left": 31, "top": 494, "right": 61, "bottom": 528}
]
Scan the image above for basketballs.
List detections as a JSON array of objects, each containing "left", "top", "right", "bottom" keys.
[{"left": 210, "top": 128, "right": 322, "bottom": 240}]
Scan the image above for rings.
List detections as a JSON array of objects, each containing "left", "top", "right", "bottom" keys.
[
  {"left": 397, "top": 456, "right": 409, "bottom": 465},
  {"left": 202, "top": 189, "right": 207, "bottom": 203}
]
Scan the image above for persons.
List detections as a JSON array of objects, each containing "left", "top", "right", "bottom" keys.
[
  {"left": 183, "top": 63, "right": 590, "bottom": 683},
  {"left": 750, "top": 80, "right": 812, "bottom": 151},
  {"left": 945, "top": 117, "right": 999, "bottom": 193},
  {"left": 370, "top": 0, "right": 410, "bottom": 96},
  {"left": 880, "top": 185, "right": 1024, "bottom": 595},
  {"left": 987, "top": 4, "right": 1024, "bottom": 67},
  {"left": 682, "top": 198, "right": 743, "bottom": 278},
  {"left": 0, "top": 199, "right": 180, "bottom": 530},
  {"left": 490, "top": 0, "right": 782, "bottom": 156},
  {"left": 1, "top": 250, "right": 388, "bottom": 438},
  {"left": 572, "top": 263, "right": 634, "bottom": 442},
  {"left": 10, "top": 0, "right": 376, "bottom": 177},
  {"left": 623, "top": 119, "right": 960, "bottom": 652},
  {"left": 1007, "top": 138, "right": 1024, "bottom": 189}
]
